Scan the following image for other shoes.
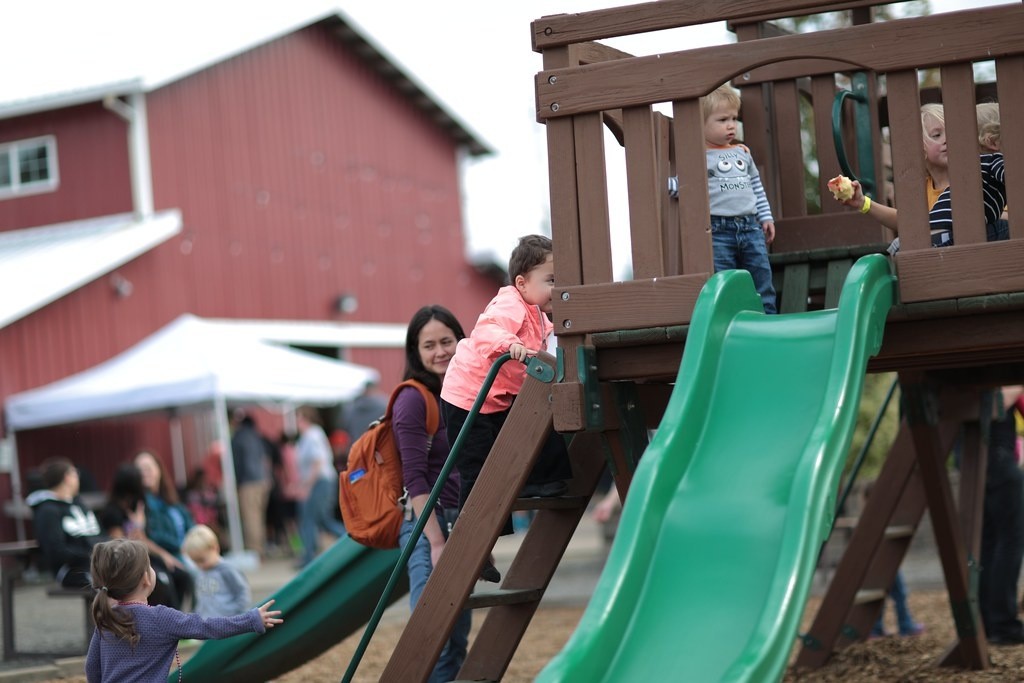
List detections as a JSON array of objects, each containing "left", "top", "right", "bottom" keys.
[
  {"left": 983, "top": 619, "right": 1024, "bottom": 645},
  {"left": 868, "top": 631, "right": 893, "bottom": 642},
  {"left": 478, "top": 559, "right": 500, "bottom": 584},
  {"left": 900, "top": 624, "right": 924, "bottom": 637}
]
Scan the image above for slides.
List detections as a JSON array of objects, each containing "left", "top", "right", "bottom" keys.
[
  {"left": 529, "top": 241, "right": 896, "bottom": 683},
  {"left": 167, "top": 527, "right": 410, "bottom": 683}
]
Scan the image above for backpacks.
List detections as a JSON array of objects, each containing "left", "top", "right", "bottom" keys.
[{"left": 338, "top": 380, "right": 439, "bottom": 550}]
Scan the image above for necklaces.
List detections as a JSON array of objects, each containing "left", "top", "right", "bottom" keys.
[{"left": 117, "top": 602, "right": 183, "bottom": 683}]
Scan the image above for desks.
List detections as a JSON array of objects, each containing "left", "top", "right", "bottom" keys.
[{"left": 7, "top": 493, "right": 110, "bottom": 523}]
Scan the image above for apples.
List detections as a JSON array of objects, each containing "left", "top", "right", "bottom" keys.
[{"left": 828, "top": 174, "right": 855, "bottom": 201}]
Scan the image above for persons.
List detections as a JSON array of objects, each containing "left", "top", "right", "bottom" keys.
[
  {"left": 863, "top": 482, "right": 924, "bottom": 636},
  {"left": 348, "top": 382, "right": 389, "bottom": 445},
  {"left": 84, "top": 537, "right": 284, "bottom": 683},
  {"left": 668, "top": 84, "right": 778, "bottom": 314},
  {"left": 833, "top": 102, "right": 1011, "bottom": 257},
  {"left": 26, "top": 447, "right": 196, "bottom": 612},
  {"left": 992, "top": 383, "right": 1024, "bottom": 468},
  {"left": 392, "top": 304, "right": 472, "bottom": 683},
  {"left": 286, "top": 403, "right": 347, "bottom": 571},
  {"left": 230, "top": 414, "right": 275, "bottom": 553},
  {"left": 183, "top": 524, "right": 251, "bottom": 620},
  {"left": 437, "top": 235, "right": 574, "bottom": 583},
  {"left": 180, "top": 469, "right": 228, "bottom": 553}
]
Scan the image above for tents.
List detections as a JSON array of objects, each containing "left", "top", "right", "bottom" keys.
[{"left": 3, "top": 310, "right": 385, "bottom": 559}]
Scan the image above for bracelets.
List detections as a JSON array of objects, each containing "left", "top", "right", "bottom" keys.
[{"left": 857, "top": 195, "right": 871, "bottom": 214}]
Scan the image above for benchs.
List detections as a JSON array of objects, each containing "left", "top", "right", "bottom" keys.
[{"left": 45, "top": 585, "right": 102, "bottom": 648}]
